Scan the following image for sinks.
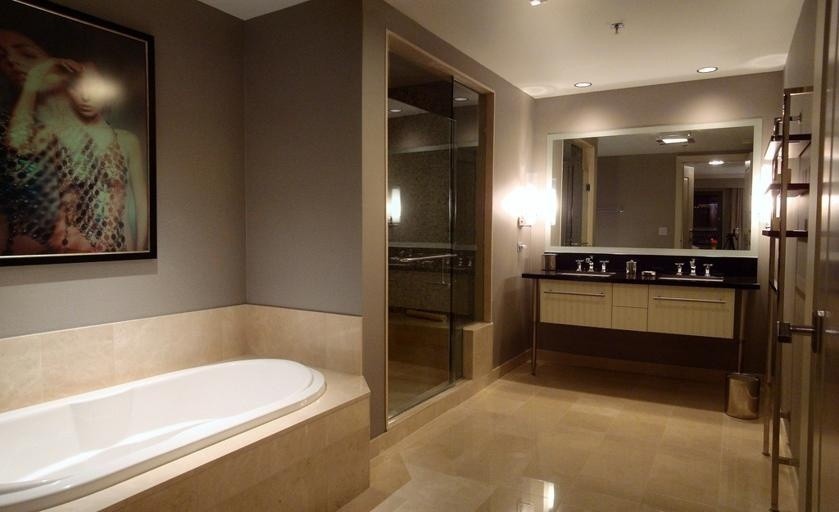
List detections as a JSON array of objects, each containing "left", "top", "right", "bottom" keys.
[
  {"left": 556, "top": 273, "right": 616, "bottom": 277},
  {"left": 659, "top": 275, "right": 724, "bottom": 282}
]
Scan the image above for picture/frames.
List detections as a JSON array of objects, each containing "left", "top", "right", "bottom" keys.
[{"left": 1, "top": 0, "right": 157, "bottom": 268}]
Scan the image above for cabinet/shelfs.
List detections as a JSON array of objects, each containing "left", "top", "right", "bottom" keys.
[
  {"left": 647, "top": 285, "right": 735, "bottom": 340},
  {"left": 389, "top": 255, "right": 473, "bottom": 317},
  {"left": 538, "top": 280, "right": 612, "bottom": 330},
  {"left": 612, "top": 284, "right": 648, "bottom": 333},
  {"left": 759, "top": 0, "right": 816, "bottom": 511}
]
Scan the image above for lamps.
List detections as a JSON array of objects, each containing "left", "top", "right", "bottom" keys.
[{"left": 387, "top": 185, "right": 404, "bottom": 227}]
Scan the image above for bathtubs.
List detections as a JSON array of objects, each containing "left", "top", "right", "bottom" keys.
[{"left": 0, "top": 358, "right": 325, "bottom": 511}]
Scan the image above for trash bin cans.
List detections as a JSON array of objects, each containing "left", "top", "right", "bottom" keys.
[{"left": 724, "top": 371, "right": 762, "bottom": 420}]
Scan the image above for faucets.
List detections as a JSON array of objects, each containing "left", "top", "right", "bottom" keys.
[
  {"left": 586, "top": 258, "right": 596, "bottom": 274},
  {"left": 690, "top": 258, "right": 697, "bottom": 277}
]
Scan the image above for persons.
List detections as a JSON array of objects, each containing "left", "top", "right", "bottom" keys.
[
  {"left": 6, "top": 56, "right": 149, "bottom": 255},
  {"left": 0, "top": 31, "right": 65, "bottom": 255}
]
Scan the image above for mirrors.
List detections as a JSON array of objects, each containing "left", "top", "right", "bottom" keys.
[{"left": 543, "top": 118, "right": 763, "bottom": 258}]
